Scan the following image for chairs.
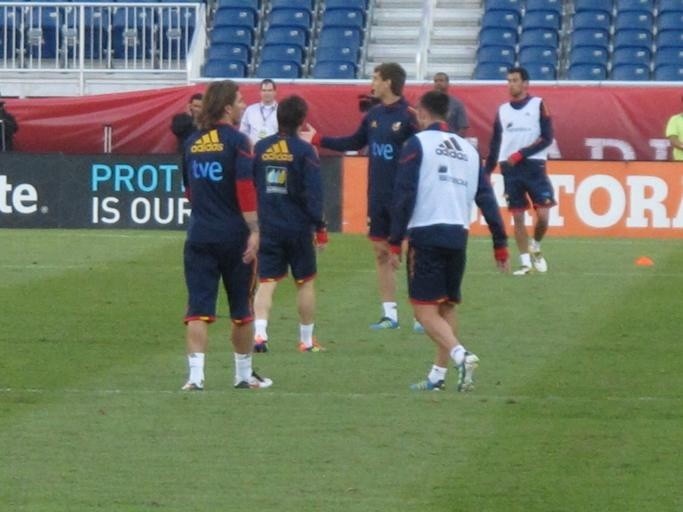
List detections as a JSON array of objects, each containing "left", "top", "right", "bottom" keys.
[{"left": 473, "top": 0, "right": 683, "bottom": 82}]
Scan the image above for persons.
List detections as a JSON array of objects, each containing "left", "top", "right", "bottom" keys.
[
  {"left": 485, "top": 69, "right": 557, "bottom": 276},
  {"left": 299, "top": 63, "right": 427, "bottom": 332},
  {"left": 239, "top": 79, "right": 278, "bottom": 146},
  {"left": 666, "top": 113, "right": 683, "bottom": 161},
  {"left": 183, "top": 81, "right": 273, "bottom": 390},
  {"left": 388, "top": 91, "right": 509, "bottom": 393},
  {"left": 171, "top": 94, "right": 203, "bottom": 189},
  {"left": 0, "top": 102, "right": 16, "bottom": 150},
  {"left": 253, "top": 97, "right": 330, "bottom": 352},
  {"left": 434, "top": 73, "right": 469, "bottom": 137}
]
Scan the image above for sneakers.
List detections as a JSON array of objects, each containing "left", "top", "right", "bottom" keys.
[
  {"left": 252, "top": 335, "right": 268, "bottom": 354},
  {"left": 235, "top": 371, "right": 273, "bottom": 390},
  {"left": 410, "top": 377, "right": 447, "bottom": 391},
  {"left": 302, "top": 341, "right": 331, "bottom": 352},
  {"left": 454, "top": 350, "right": 478, "bottom": 391},
  {"left": 369, "top": 317, "right": 425, "bottom": 332},
  {"left": 514, "top": 265, "right": 533, "bottom": 279},
  {"left": 183, "top": 374, "right": 206, "bottom": 390},
  {"left": 529, "top": 247, "right": 550, "bottom": 272}
]
